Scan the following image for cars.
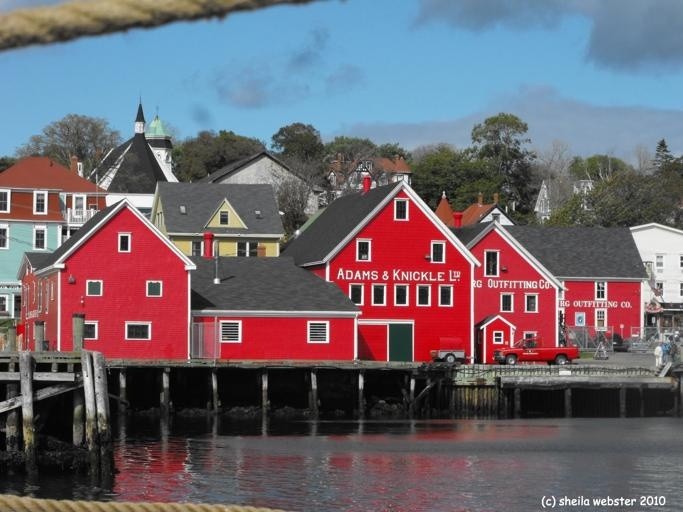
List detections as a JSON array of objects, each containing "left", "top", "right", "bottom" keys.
[{"left": 565, "top": 329, "right": 680, "bottom": 355}]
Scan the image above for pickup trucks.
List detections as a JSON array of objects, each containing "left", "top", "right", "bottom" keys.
[{"left": 492, "top": 334, "right": 580, "bottom": 365}]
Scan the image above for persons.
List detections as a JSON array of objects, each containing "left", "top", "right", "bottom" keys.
[{"left": 655, "top": 335, "right": 677, "bottom": 367}]
